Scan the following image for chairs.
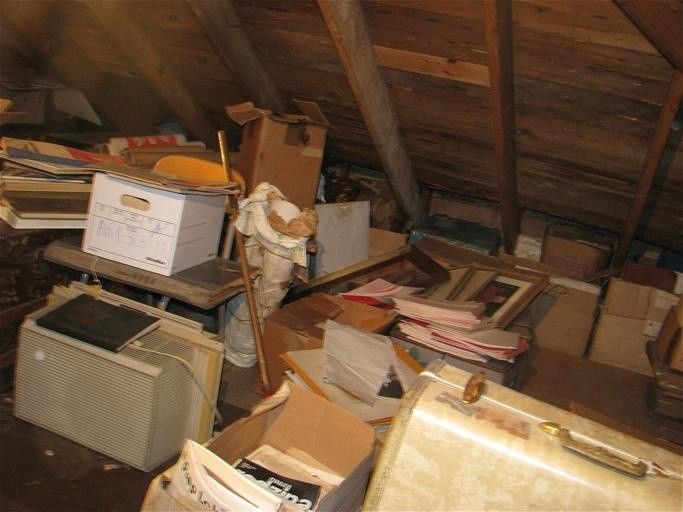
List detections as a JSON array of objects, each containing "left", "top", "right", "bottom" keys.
[{"left": 79, "top": 154, "right": 246, "bottom": 343}]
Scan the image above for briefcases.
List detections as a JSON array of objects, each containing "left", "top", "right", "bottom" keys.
[{"left": 362, "top": 359, "right": 682, "bottom": 512}]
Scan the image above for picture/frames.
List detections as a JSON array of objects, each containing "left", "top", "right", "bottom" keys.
[{"left": 409, "top": 261, "right": 550, "bottom": 330}]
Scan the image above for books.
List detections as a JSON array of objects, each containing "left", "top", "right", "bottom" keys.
[
  {"left": 167, "top": 439, "right": 346, "bottom": 511},
  {"left": 0, "top": 126, "right": 206, "bottom": 235},
  {"left": 335, "top": 262, "right": 532, "bottom": 362},
  {"left": 277, "top": 319, "right": 425, "bottom": 433}
]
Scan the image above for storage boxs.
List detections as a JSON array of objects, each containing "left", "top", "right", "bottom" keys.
[
  {"left": 139, "top": 381, "right": 376, "bottom": 512},
  {"left": 530, "top": 222, "right": 683, "bottom": 377},
  {"left": 227, "top": 99, "right": 327, "bottom": 207},
  {"left": 80, "top": 171, "right": 227, "bottom": 277},
  {"left": 311, "top": 201, "right": 370, "bottom": 277},
  {"left": 255, "top": 292, "right": 399, "bottom": 394}
]
